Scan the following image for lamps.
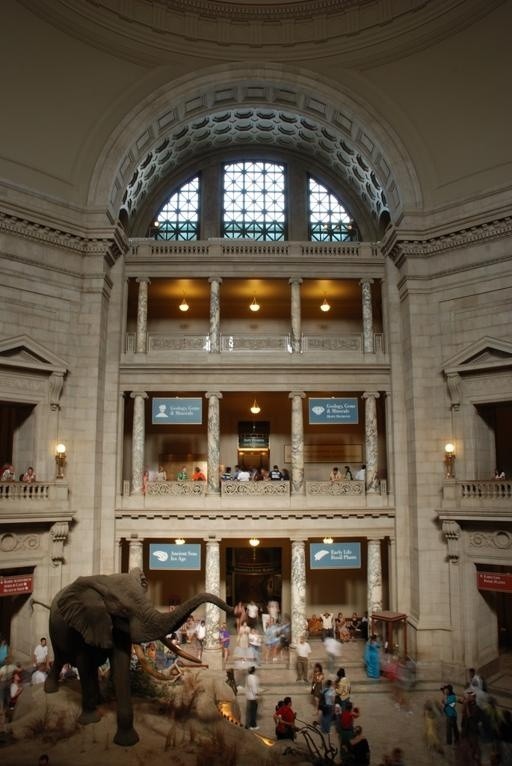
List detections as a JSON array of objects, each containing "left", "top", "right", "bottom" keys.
[
  {"left": 322, "top": 536, "right": 333, "bottom": 545},
  {"left": 249, "top": 536, "right": 259, "bottom": 547},
  {"left": 55, "top": 444, "right": 66, "bottom": 478},
  {"left": 179, "top": 295, "right": 189, "bottom": 312},
  {"left": 249, "top": 296, "right": 260, "bottom": 312},
  {"left": 320, "top": 297, "right": 331, "bottom": 312},
  {"left": 250, "top": 397, "right": 261, "bottom": 414},
  {"left": 174, "top": 538, "right": 185, "bottom": 545},
  {"left": 444, "top": 443, "right": 455, "bottom": 479}
]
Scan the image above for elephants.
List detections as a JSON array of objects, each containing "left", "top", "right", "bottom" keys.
[{"left": 29, "top": 567, "right": 236, "bottom": 747}]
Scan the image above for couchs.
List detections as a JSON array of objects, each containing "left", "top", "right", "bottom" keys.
[
  {"left": 335, "top": 618, "right": 362, "bottom": 639},
  {"left": 308, "top": 617, "right": 334, "bottom": 641}
]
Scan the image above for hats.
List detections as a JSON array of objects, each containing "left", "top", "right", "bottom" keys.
[{"left": 441, "top": 685, "right": 455, "bottom": 694}]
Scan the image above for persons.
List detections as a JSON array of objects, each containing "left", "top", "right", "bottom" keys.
[
  {"left": 32, "top": 638, "right": 52, "bottom": 685},
  {"left": 130, "top": 633, "right": 180, "bottom": 670},
  {"left": 355, "top": 465, "right": 366, "bottom": 480},
  {"left": 234, "top": 600, "right": 258, "bottom": 631},
  {"left": 60, "top": 664, "right": 80, "bottom": 680},
  {"left": 266, "top": 615, "right": 290, "bottom": 660},
  {"left": 182, "top": 615, "right": 206, "bottom": 645},
  {"left": 423, "top": 668, "right": 511, "bottom": 766},
  {"left": 220, "top": 465, "right": 289, "bottom": 490},
  {"left": 309, "top": 611, "right": 368, "bottom": 643},
  {"left": 0, "top": 463, "right": 9, "bottom": 477},
  {"left": 1, "top": 636, "right": 22, "bottom": 710},
  {"left": 328, "top": 467, "right": 341, "bottom": 481},
  {"left": 23, "top": 467, "right": 36, "bottom": 498},
  {"left": 1, "top": 464, "right": 15, "bottom": 497},
  {"left": 343, "top": 466, "right": 353, "bottom": 480},
  {"left": 273, "top": 697, "right": 296, "bottom": 741},
  {"left": 220, "top": 623, "right": 230, "bottom": 659},
  {"left": 495, "top": 467, "right": 506, "bottom": 496},
  {"left": 362, "top": 635, "right": 409, "bottom": 703},
  {"left": 237, "top": 622, "right": 261, "bottom": 666},
  {"left": 143, "top": 466, "right": 206, "bottom": 498},
  {"left": 98, "top": 657, "right": 112, "bottom": 680},
  {"left": 245, "top": 666, "right": 261, "bottom": 730},
  {"left": 291, "top": 635, "right": 371, "bottom": 766}
]
